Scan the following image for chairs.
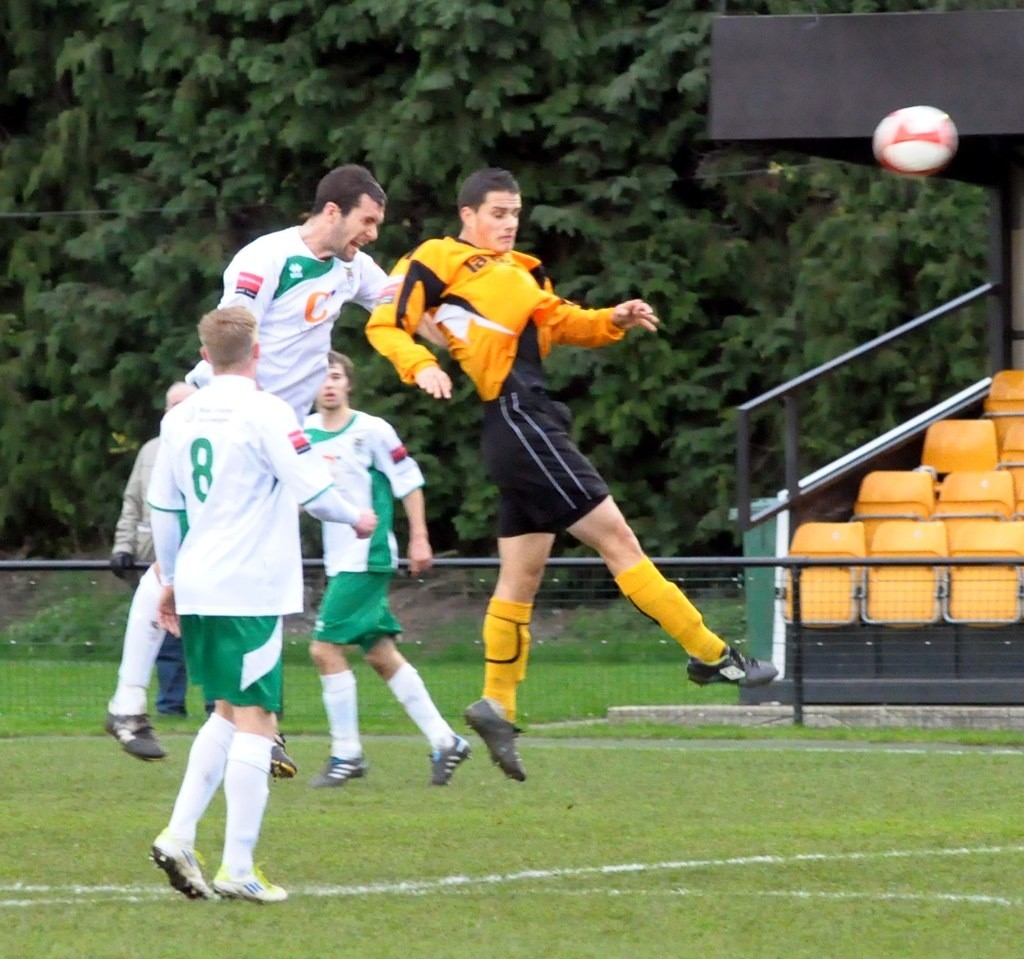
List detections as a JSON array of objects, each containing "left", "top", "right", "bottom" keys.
[{"left": 784, "top": 370, "right": 1023, "bottom": 629}]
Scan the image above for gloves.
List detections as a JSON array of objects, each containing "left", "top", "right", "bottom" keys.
[{"left": 110, "top": 552, "right": 134, "bottom": 578}]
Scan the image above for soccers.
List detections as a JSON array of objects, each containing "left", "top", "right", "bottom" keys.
[{"left": 870, "top": 104, "right": 960, "bottom": 180}]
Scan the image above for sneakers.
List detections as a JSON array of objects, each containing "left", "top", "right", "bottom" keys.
[
  {"left": 151, "top": 828, "right": 213, "bottom": 900},
  {"left": 311, "top": 753, "right": 367, "bottom": 789},
  {"left": 105, "top": 713, "right": 167, "bottom": 762},
  {"left": 428, "top": 736, "right": 471, "bottom": 785},
  {"left": 271, "top": 730, "right": 297, "bottom": 778},
  {"left": 464, "top": 697, "right": 527, "bottom": 782},
  {"left": 213, "top": 867, "right": 289, "bottom": 904},
  {"left": 686, "top": 643, "right": 779, "bottom": 689}
]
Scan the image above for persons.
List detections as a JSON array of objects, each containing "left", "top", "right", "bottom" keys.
[
  {"left": 364, "top": 167, "right": 778, "bottom": 781},
  {"left": 104, "top": 376, "right": 296, "bottom": 778},
  {"left": 145, "top": 299, "right": 377, "bottom": 902},
  {"left": 187, "top": 164, "right": 446, "bottom": 427},
  {"left": 302, "top": 350, "right": 472, "bottom": 788}
]
